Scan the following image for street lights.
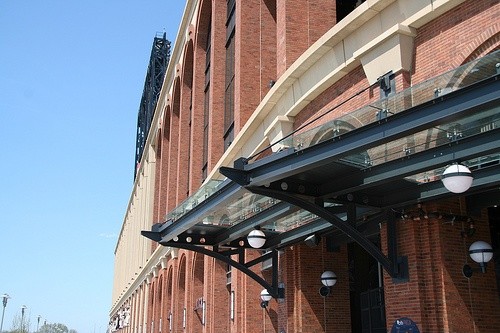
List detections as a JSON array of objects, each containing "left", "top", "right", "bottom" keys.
[
  {"left": 0, "top": 293, "right": 11, "bottom": 333},
  {"left": 20, "top": 304, "right": 27, "bottom": 333},
  {"left": 37, "top": 315, "right": 47, "bottom": 333}
]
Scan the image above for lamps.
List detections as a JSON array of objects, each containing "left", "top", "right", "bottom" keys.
[
  {"left": 247, "top": 225, "right": 267, "bottom": 249},
  {"left": 441, "top": 162, "right": 473, "bottom": 193},
  {"left": 260, "top": 289, "right": 272, "bottom": 308},
  {"left": 463, "top": 241, "right": 494, "bottom": 278},
  {"left": 319, "top": 271, "right": 337, "bottom": 297}
]
